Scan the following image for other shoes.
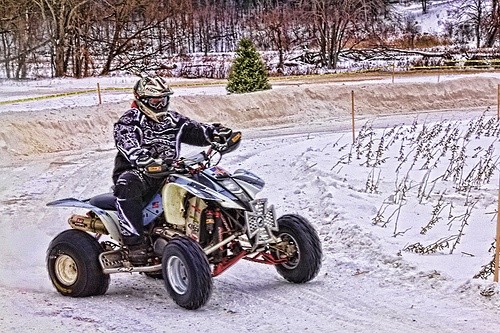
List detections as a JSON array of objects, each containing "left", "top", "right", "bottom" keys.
[{"left": 124, "top": 250, "right": 148, "bottom": 264}]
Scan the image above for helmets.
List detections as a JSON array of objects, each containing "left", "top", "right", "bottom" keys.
[{"left": 133, "top": 74, "right": 174, "bottom": 123}]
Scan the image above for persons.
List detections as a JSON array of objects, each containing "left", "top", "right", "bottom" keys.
[{"left": 112, "top": 76, "right": 233, "bottom": 263}]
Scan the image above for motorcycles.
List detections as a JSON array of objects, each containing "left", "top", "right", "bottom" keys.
[{"left": 44, "top": 131, "right": 323, "bottom": 310}]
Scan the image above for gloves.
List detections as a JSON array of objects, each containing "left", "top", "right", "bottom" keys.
[
  {"left": 205, "top": 122, "right": 233, "bottom": 144},
  {"left": 136, "top": 155, "right": 165, "bottom": 175}
]
